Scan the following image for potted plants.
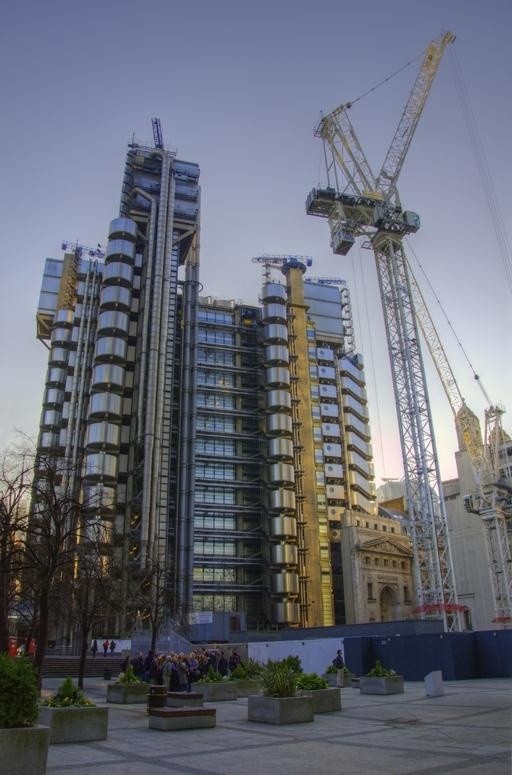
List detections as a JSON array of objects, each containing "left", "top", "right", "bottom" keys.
[
  {"left": 190, "top": 655, "right": 403, "bottom": 725},
  {"left": 2, "top": 654, "right": 150, "bottom": 775}
]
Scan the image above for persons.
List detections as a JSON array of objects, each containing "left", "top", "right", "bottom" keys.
[
  {"left": 111, "top": 640, "right": 117, "bottom": 655},
  {"left": 122, "top": 647, "right": 242, "bottom": 695},
  {"left": 332, "top": 648, "right": 344, "bottom": 688},
  {"left": 92, "top": 640, "right": 98, "bottom": 656},
  {"left": 102, "top": 640, "right": 109, "bottom": 657}
]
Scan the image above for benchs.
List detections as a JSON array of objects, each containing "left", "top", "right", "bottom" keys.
[{"left": 148, "top": 691, "right": 217, "bottom": 731}]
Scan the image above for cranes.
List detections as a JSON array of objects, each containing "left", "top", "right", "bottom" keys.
[{"left": 306, "top": 30, "right": 512, "bottom": 634}]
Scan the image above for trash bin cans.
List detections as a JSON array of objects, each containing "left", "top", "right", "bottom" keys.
[
  {"left": 146, "top": 687, "right": 168, "bottom": 715},
  {"left": 104, "top": 668, "right": 112, "bottom": 680}
]
